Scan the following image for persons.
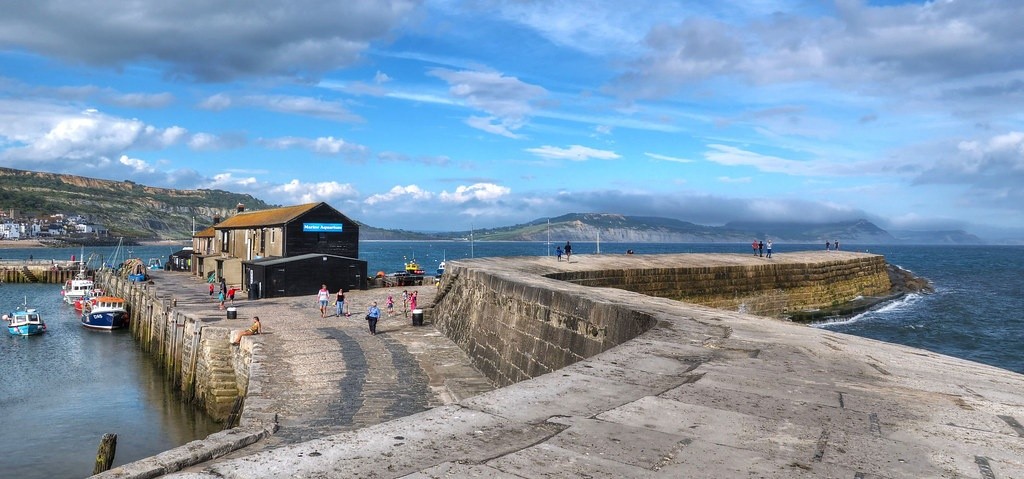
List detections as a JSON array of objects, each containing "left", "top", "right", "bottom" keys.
[
  {"left": 826, "top": 240, "right": 831, "bottom": 251},
  {"left": 402, "top": 290, "right": 418, "bottom": 316},
  {"left": 555, "top": 246, "right": 563, "bottom": 261},
  {"left": 766, "top": 239, "right": 773, "bottom": 258},
  {"left": 220, "top": 278, "right": 227, "bottom": 300},
  {"left": 317, "top": 284, "right": 329, "bottom": 317},
  {"left": 210, "top": 284, "right": 214, "bottom": 295},
  {"left": 834, "top": 240, "right": 839, "bottom": 251},
  {"left": 230, "top": 317, "right": 263, "bottom": 346},
  {"left": 367, "top": 301, "right": 381, "bottom": 335},
  {"left": 227, "top": 286, "right": 240, "bottom": 305},
  {"left": 751, "top": 240, "right": 758, "bottom": 256},
  {"left": 564, "top": 241, "right": 571, "bottom": 261},
  {"left": 334, "top": 290, "right": 345, "bottom": 316},
  {"left": 385, "top": 296, "right": 394, "bottom": 317},
  {"left": 758, "top": 241, "right": 764, "bottom": 257},
  {"left": 219, "top": 289, "right": 225, "bottom": 305}
]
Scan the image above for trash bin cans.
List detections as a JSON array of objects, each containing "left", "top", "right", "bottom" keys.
[
  {"left": 248, "top": 284, "right": 259, "bottom": 299},
  {"left": 227, "top": 307, "right": 237, "bottom": 319},
  {"left": 163, "top": 262, "right": 170, "bottom": 271},
  {"left": 412, "top": 308, "right": 423, "bottom": 326},
  {"left": 71, "top": 255, "right": 75, "bottom": 261}
]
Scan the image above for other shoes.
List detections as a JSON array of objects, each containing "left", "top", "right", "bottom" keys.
[{"left": 370, "top": 331, "right": 375, "bottom": 336}]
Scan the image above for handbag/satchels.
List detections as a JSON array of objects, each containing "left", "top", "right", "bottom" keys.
[{"left": 365, "top": 315, "right": 370, "bottom": 320}]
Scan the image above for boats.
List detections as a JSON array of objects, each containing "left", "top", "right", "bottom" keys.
[
  {"left": 146, "top": 257, "right": 163, "bottom": 270},
  {"left": 437, "top": 249, "right": 445, "bottom": 275},
  {"left": 404, "top": 253, "right": 426, "bottom": 275},
  {"left": 75, "top": 237, "right": 130, "bottom": 330},
  {"left": 60, "top": 253, "right": 99, "bottom": 304},
  {"left": 2, "top": 294, "right": 46, "bottom": 335}
]
